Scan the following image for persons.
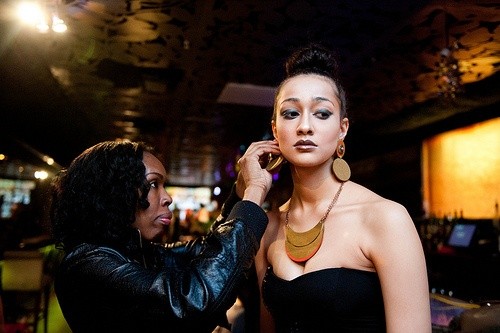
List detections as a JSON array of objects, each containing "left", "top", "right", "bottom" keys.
[
  {"left": 51, "top": 137, "right": 281, "bottom": 333},
  {"left": 211, "top": 43, "right": 432, "bottom": 333}
]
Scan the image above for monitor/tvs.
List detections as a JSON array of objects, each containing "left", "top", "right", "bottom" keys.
[{"left": 447, "top": 224, "right": 476, "bottom": 247}]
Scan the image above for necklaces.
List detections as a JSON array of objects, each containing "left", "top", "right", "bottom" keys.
[{"left": 284, "top": 179, "right": 345, "bottom": 263}]
